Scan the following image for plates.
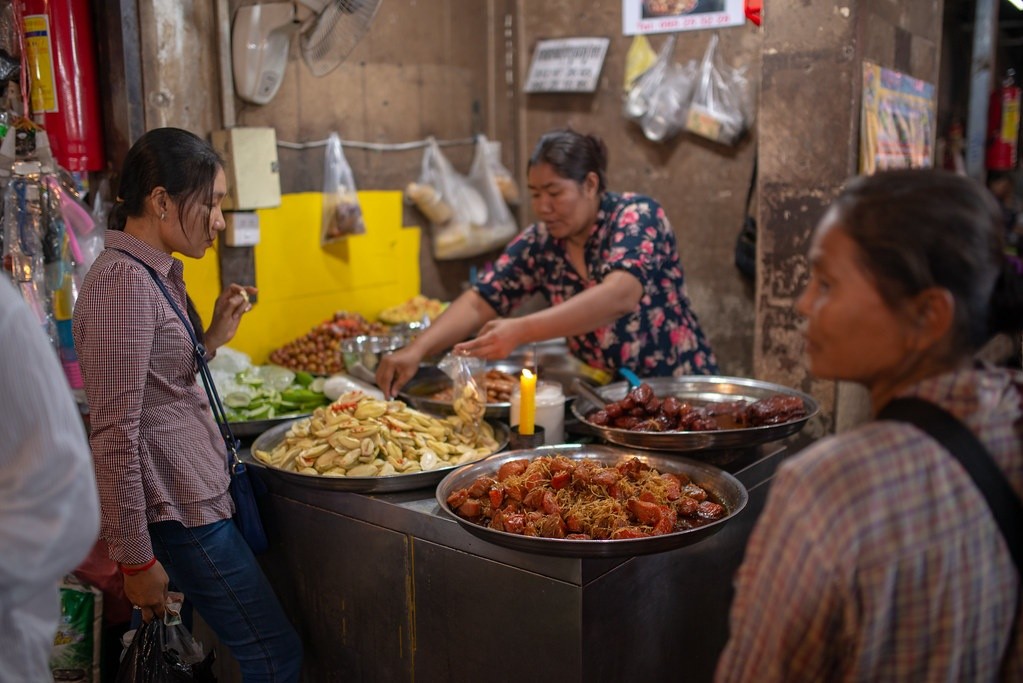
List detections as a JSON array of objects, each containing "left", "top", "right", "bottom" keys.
[
  {"left": 436, "top": 443, "right": 748, "bottom": 556},
  {"left": 395, "top": 350, "right": 602, "bottom": 418},
  {"left": 570, "top": 374, "right": 820, "bottom": 451},
  {"left": 249, "top": 410, "right": 511, "bottom": 494},
  {"left": 219, "top": 372, "right": 330, "bottom": 438}
]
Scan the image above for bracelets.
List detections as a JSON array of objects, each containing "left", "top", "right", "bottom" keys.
[{"left": 119, "top": 558, "right": 156, "bottom": 575}]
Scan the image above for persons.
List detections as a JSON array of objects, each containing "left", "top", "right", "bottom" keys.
[
  {"left": 710, "top": 166, "right": 1022, "bottom": 683},
  {"left": 0, "top": 268, "right": 99, "bottom": 683},
  {"left": 374, "top": 130, "right": 721, "bottom": 401},
  {"left": 71, "top": 126, "right": 305, "bottom": 683}
]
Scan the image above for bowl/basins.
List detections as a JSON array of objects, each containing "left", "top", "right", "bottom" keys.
[{"left": 335, "top": 336, "right": 411, "bottom": 386}]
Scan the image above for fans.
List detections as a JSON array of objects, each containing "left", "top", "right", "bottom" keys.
[{"left": 230, "top": 0, "right": 382, "bottom": 106}]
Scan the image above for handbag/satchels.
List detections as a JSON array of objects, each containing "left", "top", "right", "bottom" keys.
[
  {"left": 483, "top": 141, "right": 521, "bottom": 206},
  {"left": 419, "top": 137, "right": 487, "bottom": 245},
  {"left": 321, "top": 128, "right": 367, "bottom": 246},
  {"left": 114, "top": 616, "right": 214, "bottom": 683},
  {"left": 626, "top": 35, "right": 698, "bottom": 141},
  {"left": 229, "top": 460, "right": 269, "bottom": 553},
  {"left": 432, "top": 132, "right": 518, "bottom": 262},
  {"left": 689, "top": 33, "right": 754, "bottom": 147},
  {"left": 733, "top": 147, "right": 756, "bottom": 281}
]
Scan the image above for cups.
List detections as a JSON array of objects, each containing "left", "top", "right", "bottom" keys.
[
  {"left": 51, "top": 247, "right": 84, "bottom": 389},
  {"left": 509, "top": 380, "right": 567, "bottom": 446}
]
